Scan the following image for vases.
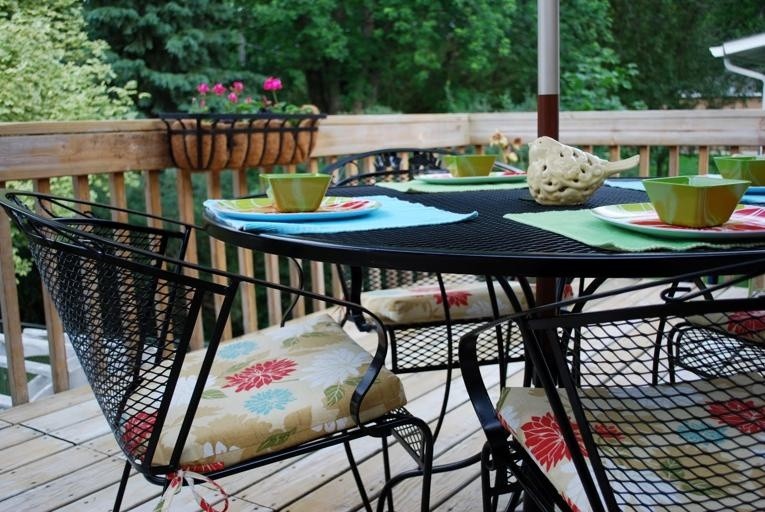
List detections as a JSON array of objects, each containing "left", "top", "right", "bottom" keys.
[{"left": 158, "top": 111, "right": 328, "bottom": 174}]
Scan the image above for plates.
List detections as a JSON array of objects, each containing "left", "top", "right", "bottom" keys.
[
  {"left": 413, "top": 171, "right": 530, "bottom": 185},
  {"left": 592, "top": 203, "right": 763, "bottom": 238},
  {"left": 746, "top": 187, "right": 765, "bottom": 195},
  {"left": 201, "top": 196, "right": 383, "bottom": 221}
]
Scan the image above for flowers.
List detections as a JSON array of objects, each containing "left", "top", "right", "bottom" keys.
[{"left": 192, "top": 74, "right": 288, "bottom": 117}]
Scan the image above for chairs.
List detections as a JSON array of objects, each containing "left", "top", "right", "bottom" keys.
[
  {"left": 0, "top": 187, "right": 435, "bottom": 511},
  {"left": 660, "top": 270, "right": 765, "bottom": 385},
  {"left": 242, "top": 145, "right": 579, "bottom": 510},
  {"left": 457, "top": 252, "right": 765, "bottom": 510}
]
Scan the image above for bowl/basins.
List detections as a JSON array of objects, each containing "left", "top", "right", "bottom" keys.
[
  {"left": 641, "top": 174, "right": 753, "bottom": 227},
  {"left": 256, "top": 171, "right": 331, "bottom": 209},
  {"left": 445, "top": 153, "right": 494, "bottom": 177},
  {"left": 711, "top": 155, "right": 765, "bottom": 184}
]
